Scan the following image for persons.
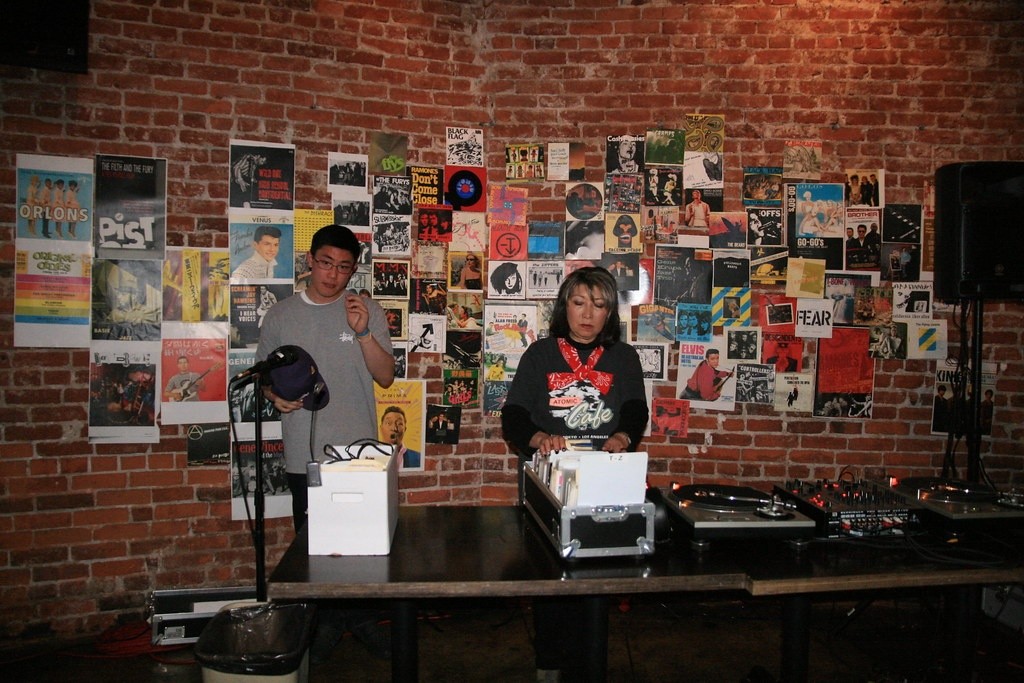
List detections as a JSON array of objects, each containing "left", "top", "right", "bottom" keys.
[
  {"left": 725, "top": 299, "right": 741, "bottom": 319},
  {"left": 817, "top": 394, "right": 871, "bottom": 419},
  {"left": 254, "top": 224, "right": 395, "bottom": 583},
  {"left": 727, "top": 330, "right": 756, "bottom": 358},
  {"left": 677, "top": 256, "right": 706, "bottom": 304},
  {"left": 855, "top": 295, "right": 875, "bottom": 320},
  {"left": 844, "top": 172, "right": 879, "bottom": 207},
  {"left": 867, "top": 325, "right": 905, "bottom": 360},
  {"left": 767, "top": 342, "right": 817, "bottom": 372},
  {"left": 501, "top": 265, "right": 649, "bottom": 457},
  {"left": 686, "top": 189, "right": 711, "bottom": 229},
  {"left": 748, "top": 211, "right": 782, "bottom": 246},
  {"left": 933, "top": 382, "right": 994, "bottom": 436},
  {"left": 889, "top": 232, "right": 920, "bottom": 282},
  {"left": 786, "top": 387, "right": 798, "bottom": 408},
  {"left": 799, "top": 192, "right": 843, "bottom": 237},
  {"left": 737, "top": 369, "right": 774, "bottom": 403},
  {"left": 846, "top": 222, "right": 881, "bottom": 268},
  {"left": 28, "top": 136, "right": 678, "bottom": 497},
  {"left": 767, "top": 306, "right": 791, "bottom": 323},
  {"left": 741, "top": 175, "right": 781, "bottom": 201},
  {"left": 679, "top": 310, "right": 711, "bottom": 336},
  {"left": 679, "top": 349, "right": 735, "bottom": 401}
]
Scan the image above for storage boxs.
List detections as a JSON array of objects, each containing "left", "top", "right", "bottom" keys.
[
  {"left": 150, "top": 585, "right": 256, "bottom": 646},
  {"left": 309, "top": 445, "right": 398, "bottom": 556},
  {"left": 520, "top": 459, "right": 654, "bottom": 563}
]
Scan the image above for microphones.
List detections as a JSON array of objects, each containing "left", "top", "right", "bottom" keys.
[{"left": 230, "top": 348, "right": 299, "bottom": 383}]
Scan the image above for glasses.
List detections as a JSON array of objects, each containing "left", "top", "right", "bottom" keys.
[
  {"left": 466, "top": 258, "right": 476, "bottom": 261},
  {"left": 311, "top": 258, "right": 356, "bottom": 274},
  {"left": 741, "top": 351, "right": 747, "bottom": 353}
]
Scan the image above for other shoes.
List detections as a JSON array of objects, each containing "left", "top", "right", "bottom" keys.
[
  {"left": 309, "top": 620, "right": 345, "bottom": 662},
  {"left": 348, "top": 618, "right": 395, "bottom": 658}
]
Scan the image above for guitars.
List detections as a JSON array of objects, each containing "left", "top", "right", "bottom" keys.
[
  {"left": 712, "top": 366, "right": 734, "bottom": 398},
  {"left": 168, "top": 360, "right": 223, "bottom": 402}
]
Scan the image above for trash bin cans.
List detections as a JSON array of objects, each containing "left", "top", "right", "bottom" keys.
[{"left": 194, "top": 602, "right": 319, "bottom": 683}]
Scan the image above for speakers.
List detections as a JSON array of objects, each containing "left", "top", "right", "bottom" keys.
[{"left": 933, "top": 161, "right": 1024, "bottom": 305}]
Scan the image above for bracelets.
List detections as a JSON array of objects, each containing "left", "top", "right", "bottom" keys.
[
  {"left": 356, "top": 331, "right": 372, "bottom": 343},
  {"left": 355, "top": 328, "right": 369, "bottom": 336}
]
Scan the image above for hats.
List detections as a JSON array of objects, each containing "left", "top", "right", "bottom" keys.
[{"left": 777, "top": 343, "right": 789, "bottom": 349}]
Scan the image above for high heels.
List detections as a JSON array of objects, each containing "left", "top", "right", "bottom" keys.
[{"left": 32, "top": 231, "right": 78, "bottom": 240}]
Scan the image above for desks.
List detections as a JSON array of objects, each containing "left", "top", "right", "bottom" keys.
[{"left": 268, "top": 507, "right": 1020, "bottom": 683}]
